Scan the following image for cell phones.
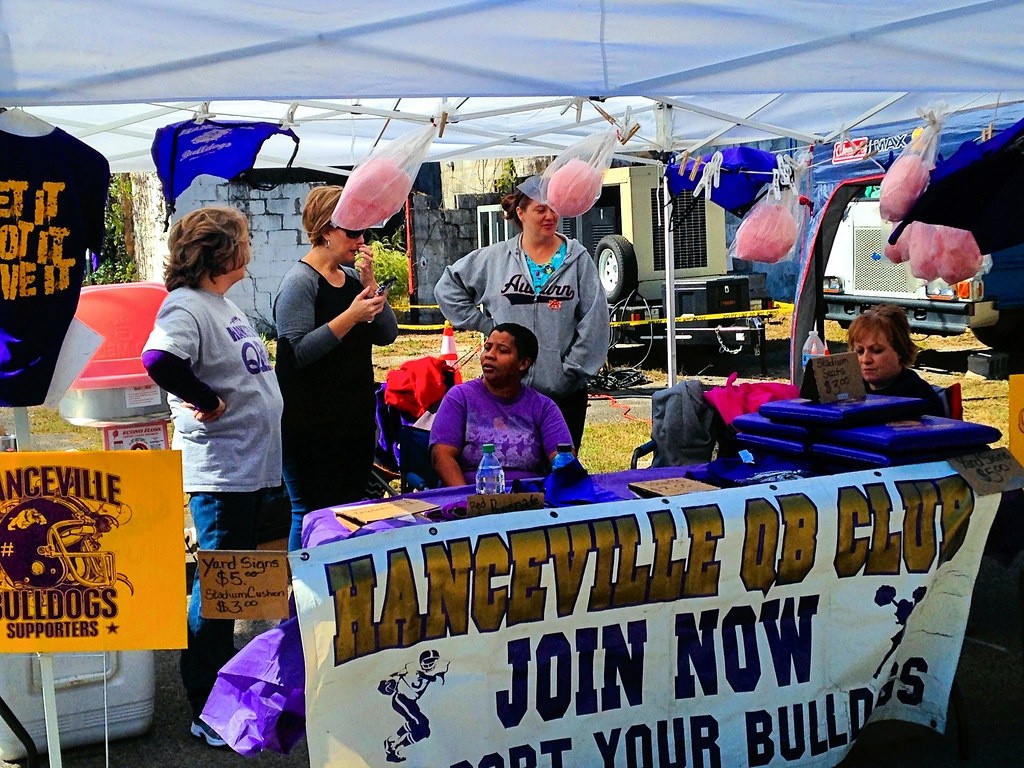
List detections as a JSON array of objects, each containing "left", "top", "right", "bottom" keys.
[{"left": 374, "top": 277, "right": 398, "bottom": 298}]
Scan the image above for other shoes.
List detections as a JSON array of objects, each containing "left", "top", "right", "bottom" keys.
[{"left": 191, "top": 716, "right": 226, "bottom": 745}]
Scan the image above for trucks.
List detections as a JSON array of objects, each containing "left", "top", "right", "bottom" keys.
[{"left": 475, "top": 167, "right": 777, "bottom": 367}]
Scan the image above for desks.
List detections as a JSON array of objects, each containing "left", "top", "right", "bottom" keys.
[{"left": 296, "top": 464, "right": 1000, "bottom": 768}]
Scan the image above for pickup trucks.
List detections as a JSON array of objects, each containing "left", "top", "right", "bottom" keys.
[{"left": 820, "top": 196, "right": 1024, "bottom": 348}]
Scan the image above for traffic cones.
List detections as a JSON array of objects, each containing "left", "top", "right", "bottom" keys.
[{"left": 440, "top": 327, "right": 462, "bottom": 387}]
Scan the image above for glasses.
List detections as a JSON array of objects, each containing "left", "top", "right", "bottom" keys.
[{"left": 329, "top": 220, "right": 366, "bottom": 239}]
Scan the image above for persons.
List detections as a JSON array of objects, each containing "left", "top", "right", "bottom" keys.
[
  {"left": 846, "top": 302, "right": 946, "bottom": 417},
  {"left": 433, "top": 188, "right": 610, "bottom": 458},
  {"left": 269, "top": 183, "right": 399, "bottom": 557},
  {"left": 427, "top": 323, "right": 575, "bottom": 490},
  {"left": 139, "top": 208, "right": 293, "bottom": 748}
]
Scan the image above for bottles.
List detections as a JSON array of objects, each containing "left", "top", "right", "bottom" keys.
[
  {"left": 477, "top": 443, "right": 506, "bottom": 494},
  {"left": 552, "top": 444, "right": 573, "bottom": 471},
  {"left": 802, "top": 331, "right": 824, "bottom": 371}
]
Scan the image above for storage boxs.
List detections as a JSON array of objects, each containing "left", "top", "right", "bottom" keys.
[
  {"left": 60, "top": 279, "right": 187, "bottom": 432},
  {"left": 0, "top": 640, "right": 152, "bottom": 766}
]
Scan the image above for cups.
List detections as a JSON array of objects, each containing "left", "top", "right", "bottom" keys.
[{"left": 0, "top": 435, "right": 17, "bottom": 450}]
